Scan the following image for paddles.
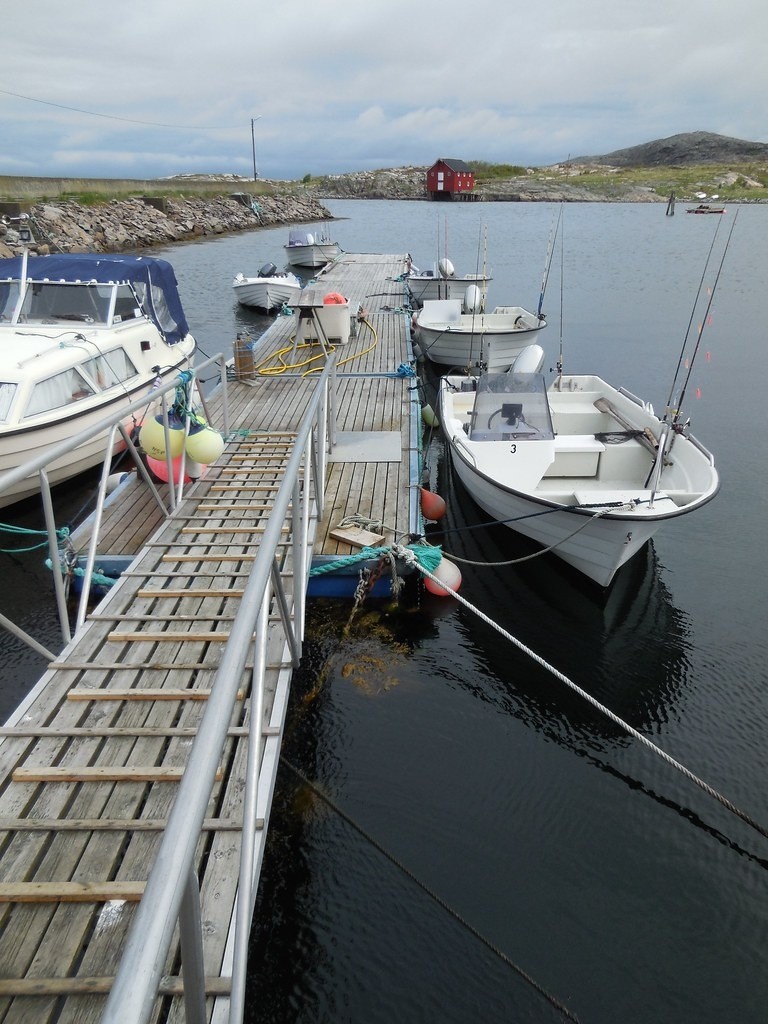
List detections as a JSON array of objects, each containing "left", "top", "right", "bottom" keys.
[{"left": 593, "top": 398, "right": 674, "bottom": 465}]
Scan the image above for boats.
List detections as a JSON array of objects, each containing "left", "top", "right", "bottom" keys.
[
  {"left": 415, "top": 284, "right": 548, "bottom": 390},
  {"left": 686, "top": 205, "right": 726, "bottom": 215},
  {"left": 282, "top": 226, "right": 344, "bottom": 269},
  {"left": 0, "top": 252, "right": 199, "bottom": 512},
  {"left": 437, "top": 343, "right": 721, "bottom": 611},
  {"left": 406, "top": 258, "right": 493, "bottom": 313},
  {"left": 233, "top": 261, "right": 302, "bottom": 315}
]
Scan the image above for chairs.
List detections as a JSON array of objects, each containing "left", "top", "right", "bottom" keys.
[{"left": 40, "top": 286, "right": 106, "bottom": 320}]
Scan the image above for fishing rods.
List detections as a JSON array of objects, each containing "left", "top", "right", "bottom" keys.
[
  {"left": 438, "top": 154, "right": 571, "bottom": 392},
  {"left": 644, "top": 206, "right": 739, "bottom": 489},
  {"left": 325, "top": 219, "right": 331, "bottom": 241}
]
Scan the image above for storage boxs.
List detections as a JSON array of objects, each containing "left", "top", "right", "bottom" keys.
[{"left": 294, "top": 298, "right": 351, "bottom": 346}]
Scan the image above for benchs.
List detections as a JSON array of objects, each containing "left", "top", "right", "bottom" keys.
[
  {"left": 543, "top": 436, "right": 605, "bottom": 477},
  {"left": 432, "top": 325, "right": 514, "bottom": 331},
  {"left": 453, "top": 403, "right": 608, "bottom": 436}
]
